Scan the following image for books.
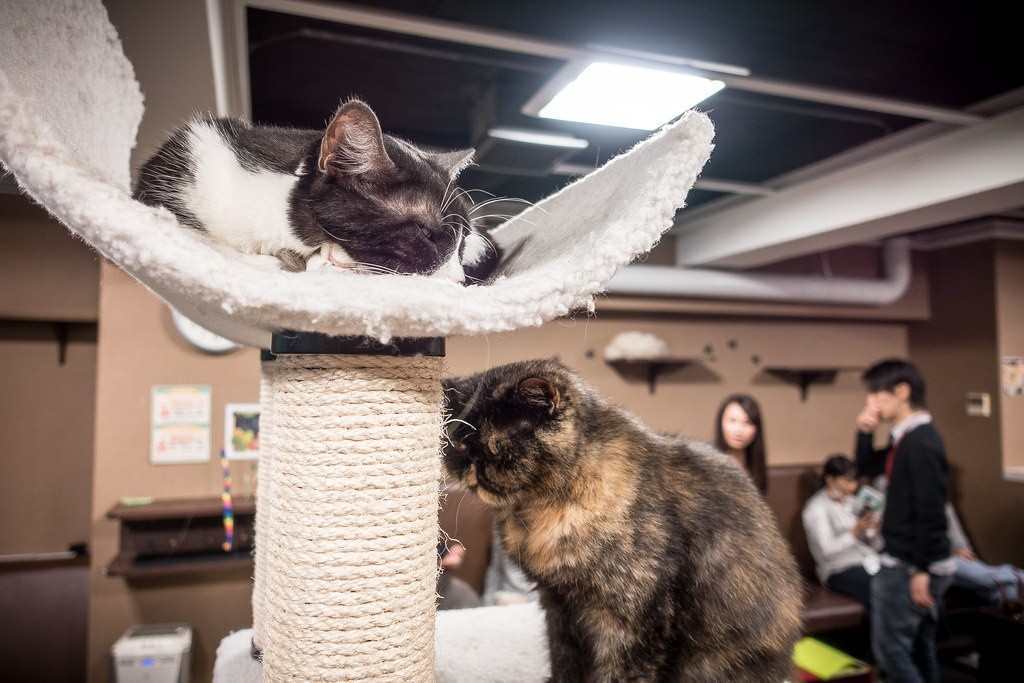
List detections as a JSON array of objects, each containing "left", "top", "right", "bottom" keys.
[
  {"left": 851, "top": 485, "right": 886, "bottom": 518},
  {"left": 793, "top": 637, "right": 870, "bottom": 680}
]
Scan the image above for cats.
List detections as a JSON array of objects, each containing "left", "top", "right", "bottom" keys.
[
  {"left": 130, "top": 99, "right": 504, "bottom": 287},
  {"left": 442, "top": 358, "right": 805, "bottom": 683}
]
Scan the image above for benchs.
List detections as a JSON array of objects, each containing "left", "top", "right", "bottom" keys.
[{"left": 756, "top": 464, "right": 874, "bottom": 627}]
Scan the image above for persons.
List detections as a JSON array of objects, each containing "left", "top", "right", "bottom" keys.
[
  {"left": 803, "top": 358, "right": 1024, "bottom": 683},
  {"left": 714, "top": 393, "right": 769, "bottom": 493}
]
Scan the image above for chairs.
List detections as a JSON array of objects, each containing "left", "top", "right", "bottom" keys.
[{"left": 433, "top": 479, "right": 499, "bottom": 609}]
